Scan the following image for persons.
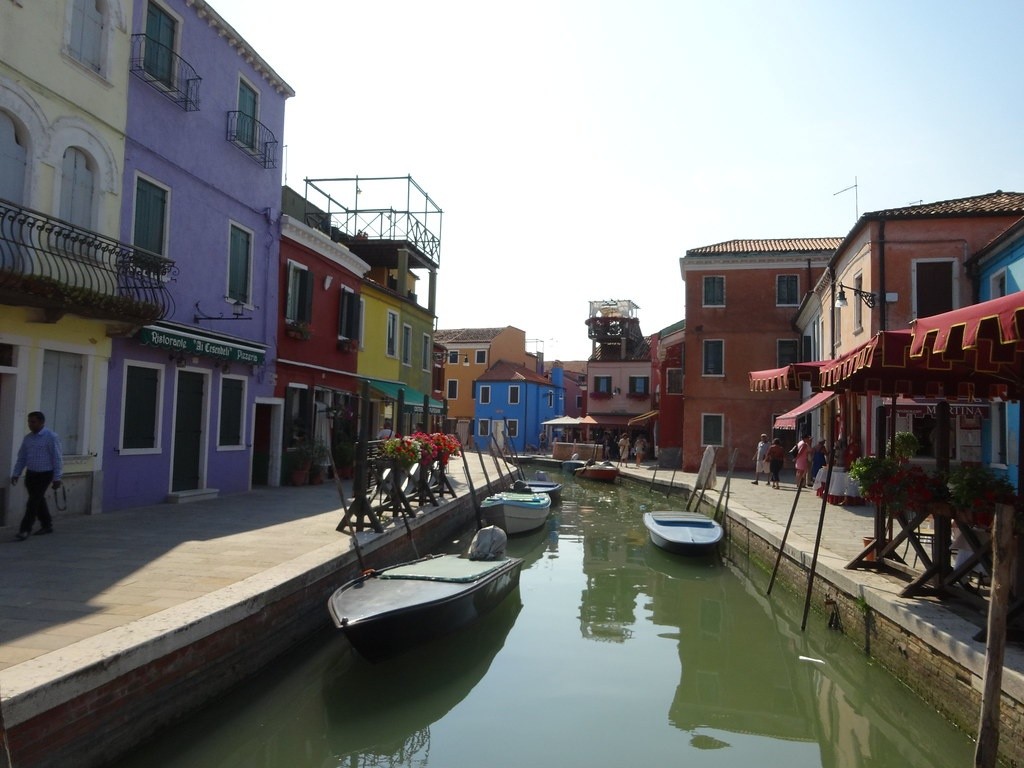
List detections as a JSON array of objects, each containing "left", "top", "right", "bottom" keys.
[
  {"left": 414, "top": 422, "right": 423, "bottom": 431},
  {"left": 11, "top": 412, "right": 63, "bottom": 538},
  {"left": 537, "top": 430, "right": 546, "bottom": 455},
  {"left": 377, "top": 421, "right": 395, "bottom": 440},
  {"left": 751, "top": 434, "right": 771, "bottom": 485},
  {"left": 765, "top": 438, "right": 784, "bottom": 489},
  {"left": 553, "top": 432, "right": 643, "bottom": 467},
  {"left": 792, "top": 434, "right": 815, "bottom": 488},
  {"left": 812, "top": 439, "right": 830, "bottom": 477}
]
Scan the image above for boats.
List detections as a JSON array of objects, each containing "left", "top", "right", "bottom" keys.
[
  {"left": 574, "top": 458, "right": 619, "bottom": 482},
  {"left": 325, "top": 524, "right": 526, "bottom": 664},
  {"left": 481, "top": 478, "right": 551, "bottom": 535},
  {"left": 533, "top": 457, "right": 564, "bottom": 468},
  {"left": 642, "top": 509, "right": 724, "bottom": 558},
  {"left": 561, "top": 453, "right": 583, "bottom": 475},
  {"left": 505, "top": 454, "right": 533, "bottom": 465},
  {"left": 509, "top": 471, "right": 564, "bottom": 501}
]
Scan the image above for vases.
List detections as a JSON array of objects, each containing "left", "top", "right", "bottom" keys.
[
  {"left": 863, "top": 537, "right": 891, "bottom": 562},
  {"left": 288, "top": 331, "right": 311, "bottom": 340}
]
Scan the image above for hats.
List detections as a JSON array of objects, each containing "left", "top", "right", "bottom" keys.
[
  {"left": 761, "top": 434, "right": 767, "bottom": 436},
  {"left": 818, "top": 437, "right": 827, "bottom": 442}
]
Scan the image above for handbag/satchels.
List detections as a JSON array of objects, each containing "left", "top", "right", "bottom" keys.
[{"left": 790, "top": 445, "right": 798, "bottom": 458}]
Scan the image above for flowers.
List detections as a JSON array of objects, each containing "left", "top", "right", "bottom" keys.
[
  {"left": 287, "top": 320, "right": 314, "bottom": 340},
  {"left": 382, "top": 432, "right": 461, "bottom": 467},
  {"left": 336, "top": 338, "right": 358, "bottom": 352}
]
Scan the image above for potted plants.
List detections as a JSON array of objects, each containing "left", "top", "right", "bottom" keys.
[
  {"left": 286, "top": 431, "right": 329, "bottom": 486},
  {"left": 332, "top": 440, "right": 357, "bottom": 481},
  {"left": 847, "top": 432, "right": 1019, "bottom": 531}
]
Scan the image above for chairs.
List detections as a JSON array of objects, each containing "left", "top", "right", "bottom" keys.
[{"left": 901, "top": 508, "right": 934, "bottom": 569}]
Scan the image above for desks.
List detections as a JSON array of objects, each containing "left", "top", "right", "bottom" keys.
[{"left": 813, "top": 465, "right": 864, "bottom": 507}]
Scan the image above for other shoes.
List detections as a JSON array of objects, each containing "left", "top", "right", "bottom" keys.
[
  {"left": 15, "top": 531, "right": 30, "bottom": 539},
  {"left": 34, "top": 527, "right": 53, "bottom": 535},
  {"left": 751, "top": 481, "right": 758, "bottom": 485}
]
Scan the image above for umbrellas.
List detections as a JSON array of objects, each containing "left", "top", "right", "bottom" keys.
[
  {"left": 539, "top": 415, "right": 584, "bottom": 442},
  {"left": 748, "top": 360, "right": 896, "bottom": 540},
  {"left": 909, "top": 289, "right": 1024, "bottom": 364},
  {"left": 818, "top": 331, "right": 1024, "bottom": 493}
]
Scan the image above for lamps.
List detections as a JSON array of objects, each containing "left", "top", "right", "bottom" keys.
[
  {"left": 193, "top": 300, "right": 252, "bottom": 324},
  {"left": 431, "top": 351, "right": 470, "bottom": 367},
  {"left": 613, "top": 386, "right": 622, "bottom": 396},
  {"left": 835, "top": 281, "right": 876, "bottom": 310}
]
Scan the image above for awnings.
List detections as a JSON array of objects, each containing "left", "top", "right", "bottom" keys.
[
  {"left": 628, "top": 410, "right": 659, "bottom": 426},
  {"left": 370, "top": 381, "right": 449, "bottom": 414},
  {"left": 138, "top": 324, "right": 266, "bottom": 366},
  {"left": 773, "top": 389, "right": 847, "bottom": 430},
  {"left": 588, "top": 415, "right": 649, "bottom": 429}
]
